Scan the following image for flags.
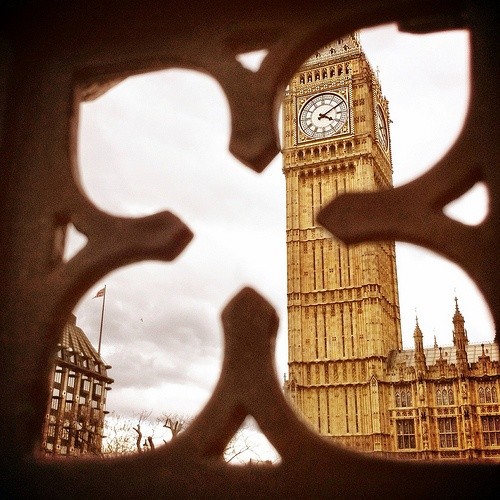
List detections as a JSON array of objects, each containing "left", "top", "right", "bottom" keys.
[{"left": 93, "top": 288, "right": 105, "bottom": 298}]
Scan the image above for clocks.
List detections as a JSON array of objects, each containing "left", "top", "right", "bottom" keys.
[
  {"left": 297, "top": 91, "right": 349, "bottom": 141},
  {"left": 375, "top": 101, "right": 391, "bottom": 153}
]
